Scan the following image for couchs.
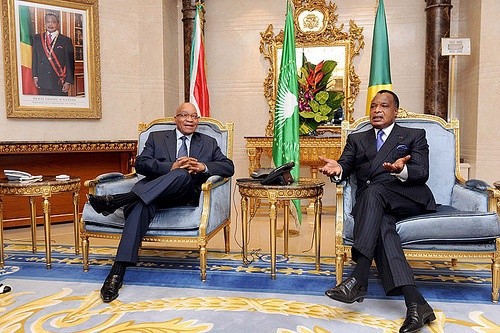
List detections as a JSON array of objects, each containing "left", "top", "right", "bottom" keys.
[
  {"left": 333, "top": 107, "right": 500, "bottom": 303},
  {"left": 80, "top": 117, "right": 235, "bottom": 282}
]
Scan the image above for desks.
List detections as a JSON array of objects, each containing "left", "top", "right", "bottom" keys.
[
  {"left": 0, "top": 176, "right": 82, "bottom": 271},
  {"left": 243, "top": 135, "right": 343, "bottom": 218},
  {"left": 236, "top": 177, "right": 325, "bottom": 280}
]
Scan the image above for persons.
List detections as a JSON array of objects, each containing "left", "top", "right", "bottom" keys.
[
  {"left": 317, "top": 89, "right": 436, "bottom": 332},
  {"left": 86, "top": 101, "right": 235, "bottom": 301},
  {"left": 32, "top": 13, "right": 75, "bottom": 96}
]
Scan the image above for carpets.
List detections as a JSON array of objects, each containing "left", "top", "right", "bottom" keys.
[{"left": 0, "top": 239, "right": 500, "bottom": 333}]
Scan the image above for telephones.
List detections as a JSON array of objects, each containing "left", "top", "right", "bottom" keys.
[
  {"left": 3, "top": 169, "right": 43, "bottom": 183},
  {"left": 260, "top": 161, "right": 295, "bottom": 185}
]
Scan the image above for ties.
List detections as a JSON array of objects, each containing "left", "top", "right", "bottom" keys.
[
  {"left": 178, "top": 136, "right": 188, "bottom": 159},
  {"left": 47, "top": 34, "right": 52, "bottom": 44},
  {"left": 376, "top": 130, "right": 385, "bottom": 152}
]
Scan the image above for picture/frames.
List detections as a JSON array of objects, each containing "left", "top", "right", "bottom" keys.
[
  {"left": 258, "top": 0, "right": 365, "bottom": 139},
  {"left": 1, "top": 1, "right": 103, "bottom": 120}
]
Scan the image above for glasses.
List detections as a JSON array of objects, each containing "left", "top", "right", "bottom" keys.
[{"left": 176, "top": 113, "right": 201, "bottom": 120}]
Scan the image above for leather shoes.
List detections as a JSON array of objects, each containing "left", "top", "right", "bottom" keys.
[
  {"left": 88, "top": 194, "right": 115, "bottom": 216},
  {"left": 400, "top": 302, "right": 436, "bottom": 333},
  {"left": 100, "top": 274, "right": 124, "bottom": 302},
  {"left": 325, "top": 276, "right": 368, "bottom": 304}
]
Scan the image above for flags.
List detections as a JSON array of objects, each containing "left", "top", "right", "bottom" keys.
[
  {"left": 189, "top": 4, "right": 211, "bottom": 118},
  {"left": 365, "top": 0, "right": 393, "bottom": 116},
  {"left": 271, "top": 0, "right": 302, "bottom": 227}
]
